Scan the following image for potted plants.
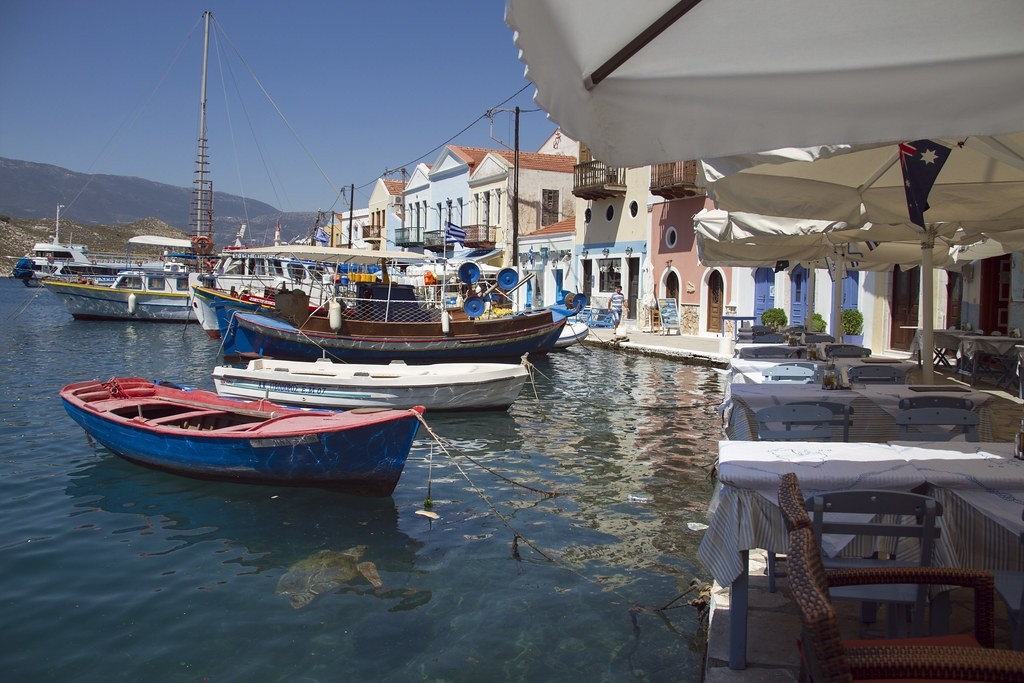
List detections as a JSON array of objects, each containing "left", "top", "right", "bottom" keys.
[{"left": 840, "top": 308, "right": 864, "bottom": 346}]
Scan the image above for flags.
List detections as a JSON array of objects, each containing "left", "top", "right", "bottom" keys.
[
  {"left": 313, "top": 228, "right": 330, "bottom": 243},
  {"left": 446, "top": 222, "right": 466, "bottom": 248},
  {"left": 898, "top": 139, "right": 954, "bottom": 227}
]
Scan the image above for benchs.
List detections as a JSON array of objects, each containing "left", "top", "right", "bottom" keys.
[
  {"left": 209, "top": 422, "right": 264, "bottom": 433},
  {"left": 144, "top": 410, "right": 227, "bottom": 425}
]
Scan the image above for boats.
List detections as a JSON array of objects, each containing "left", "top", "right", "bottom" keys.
[
  {"left": 39, "top": 270, "right": 198, "bottom": 322},
  {"left": 4, "top": 202, "right": 191, "bottom": 285},
  {"left": 60, "top": 376, "right": 426, "bottom": 497},
  {"left": 210, "top": 358, "right": 529, "bottom": 413},
  {"left": 188, "top": 246, "right": 588, "bottom": 362}
]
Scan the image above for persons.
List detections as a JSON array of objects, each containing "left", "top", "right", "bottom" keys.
[
  {"left": 427, "top": 280, "right": 485, "bottom": 320},
  {"left": 77, "top": 273, "right": 87, "bottom": 284},
  {"left": 608, "top": 286, "right": 630, "bottom": 335}
]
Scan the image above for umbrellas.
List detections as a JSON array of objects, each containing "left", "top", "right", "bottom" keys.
[{"left": 504, "top": 0, "right": 1024, "bottom": 386}]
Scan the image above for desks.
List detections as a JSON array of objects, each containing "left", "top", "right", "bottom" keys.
[{"left": 718, "top": 326, "right": 1024, "bottom": 670}]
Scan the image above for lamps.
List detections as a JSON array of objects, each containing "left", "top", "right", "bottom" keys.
[
  {"left": 625, "top": 246, "right": 633, "bottom": 256},
  {"left": 602, "top": 248, "right": 610, "bottom": 257},
  {"left": 581, "top": 250, "right": 588, "bottom": 257}
]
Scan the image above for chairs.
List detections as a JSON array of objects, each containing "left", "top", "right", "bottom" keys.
[
  {"left": 579, "top": 310, "right": 616, "bottom": 330},
  {"left": 750, "top": 323, "right": 1024, "bottom": 683}
]
